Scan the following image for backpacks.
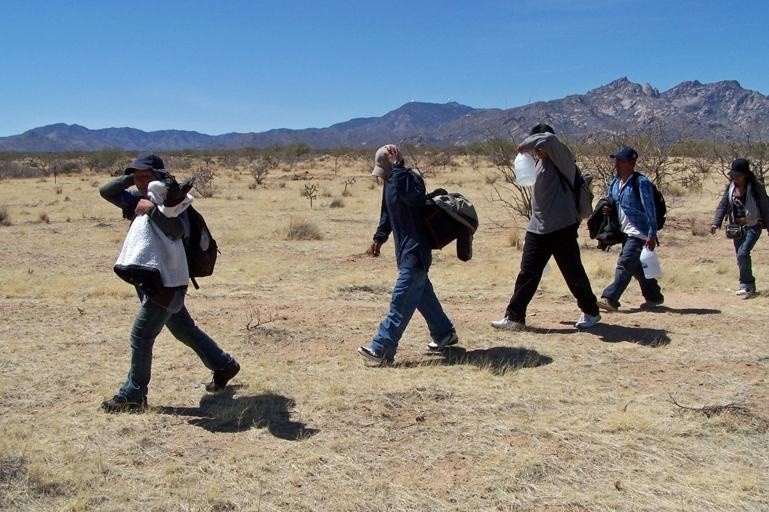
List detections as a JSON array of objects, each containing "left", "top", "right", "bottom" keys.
[
  {"left": 634, "top": 172, "right": 666, "bottom": 230},
  {"left": 553, "top": 163, "right": 594, "bottom": 218},
  {"left": 186, "top": 205, "right": 221, "bottom": 289},
  {"left": 424, "top": 189, "right": 478, "bottom": 261}
]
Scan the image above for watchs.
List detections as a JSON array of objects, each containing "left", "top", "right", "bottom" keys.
[{"left": 393, "top": 161, "right": 401, "bottom": 166}]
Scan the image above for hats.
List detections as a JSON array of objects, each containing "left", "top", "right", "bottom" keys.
[
  {"left": 124, "top": 155, "right": 164, "bottom": 174},
  {"left": 728, "top": 159, "right": 749, "bottom": 176},
  {"left": 372, "top": 144, "right": 402, "bottom": 176},
  {"left": 610, "top": 147, "right": 638, "bottom": 160}
]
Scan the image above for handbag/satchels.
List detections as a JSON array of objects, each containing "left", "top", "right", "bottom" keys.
[{"left": 726, "top": 225, "right": 743, "bottom": 239}]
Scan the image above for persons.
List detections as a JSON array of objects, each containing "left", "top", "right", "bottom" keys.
[
  {"left": 357, "top": 146, "right": 459, "bottom": 364},
  {"left": 99, "top": 156, "right": 240, "bottom": 412},
  {"left": 491, "top": 125, "right": 601, "bottom": 330},
  {"left": 596, "top": 148, "right": 664, "bottom": 311},
  {"left": 709, "top": 159, "right": 769, "bottom": 300}
]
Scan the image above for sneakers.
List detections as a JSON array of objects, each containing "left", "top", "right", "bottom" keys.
[
  {"left": 427, "top": 329, "right": 458, "bottom": 350},
  {"left": 575, "top": 312, "right": 601, "bottom": 328},
  {"left": 596, "top": 297, "right": 618, "bottom": 311},
  {"left": 490, "top": 316, "right": 525, "bottom": 328},
  {"left": 101, "top": 395, "right": 147, "bottom": 410},
  {"left": 206, "top": 358, "right": 239, "bottom": 390},
  {"left": 736, "top": 287, "right": 755, "bottom": 299},
  {"left": 358, "top": 346, "right": 393, "bottom": 363},
  {"left": 640, "top": 298, "right": 664, "bottom": 308}
]
